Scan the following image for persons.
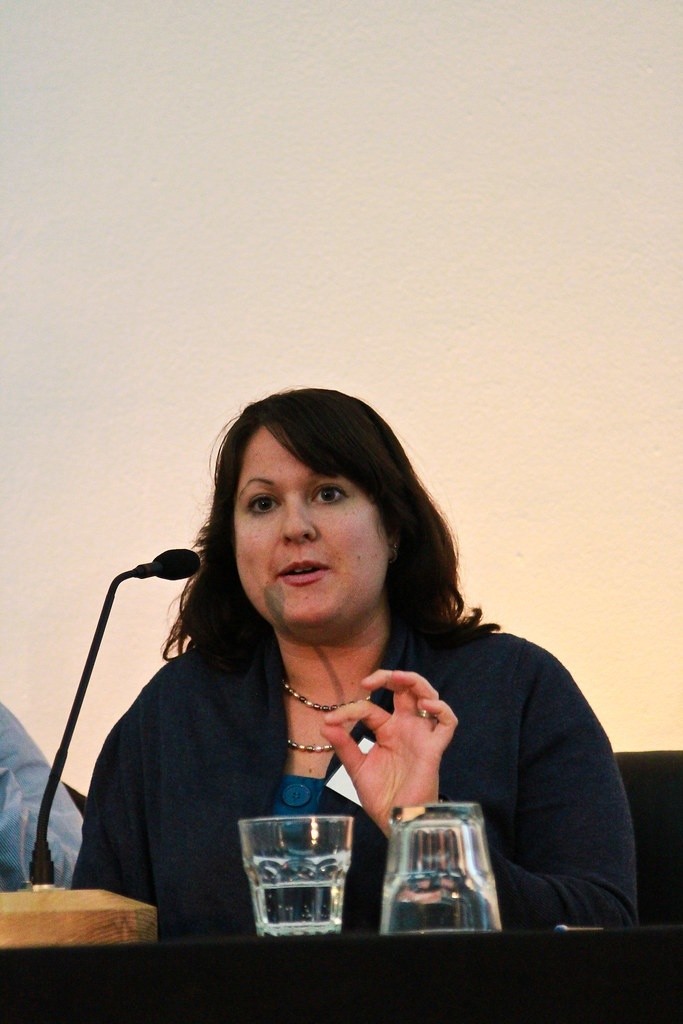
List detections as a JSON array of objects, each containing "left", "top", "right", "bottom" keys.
[
  {"left": 0, "top": 703, "right": 85, "bottom": 892},
  {"left": 78, "top": 389, "right": 637, "bottom": 939}
]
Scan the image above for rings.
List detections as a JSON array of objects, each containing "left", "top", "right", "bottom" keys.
[{"left": 416, "top": 708, "right": 437, "bottom": 718}]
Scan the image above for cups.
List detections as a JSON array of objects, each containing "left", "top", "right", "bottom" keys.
[
  {"left": 378, "top": 802, "right": 503, "bottom": 932},
  {"left": 236, "top": 813, "right": 357, "bottom": 936}
]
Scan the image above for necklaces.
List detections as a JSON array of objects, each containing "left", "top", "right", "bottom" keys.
[
  {"left": 282, "top": 680, "right": 371, "bottom": 711},
  {"left": 287, "top": 739, "right": 334, "bottom": 752}
]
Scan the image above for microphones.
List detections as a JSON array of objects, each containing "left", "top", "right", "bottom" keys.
[{"left": 21, "top": 550, "right": 203, "bottom": 886}]
